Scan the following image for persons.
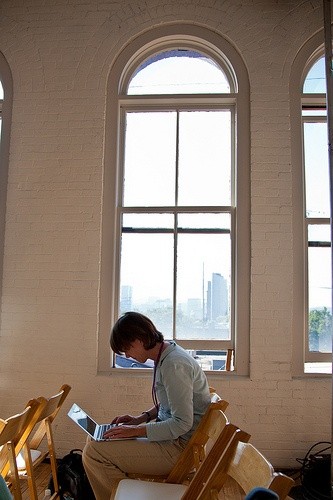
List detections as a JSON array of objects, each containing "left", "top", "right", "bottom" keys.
[{"left": 81, "top": 311, "right": 210, "bottom": 500}]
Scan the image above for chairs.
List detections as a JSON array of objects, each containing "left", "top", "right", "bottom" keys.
[
  {"left": 1, "top": 385, "right": 72, "bottom": 500},
  {"left": 109, "top": 405, "right": 241, "bottom": 500},
  {"left": 123, "top": 387, "right": 230, "bottom": 483},
  {"left": 193, "top": 426, "right": 294, "bottom": 499},
  {"left": 0, "top": 397, "right": 41, "bottom": 500}
]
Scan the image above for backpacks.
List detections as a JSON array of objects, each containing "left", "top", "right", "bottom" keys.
[{"left": 47, "top": 449, "right": 97, "bottom": 500}]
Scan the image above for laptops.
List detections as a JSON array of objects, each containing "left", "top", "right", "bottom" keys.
[{"left": 67, "top": 402, "right": 147, "bottom": 442}]
